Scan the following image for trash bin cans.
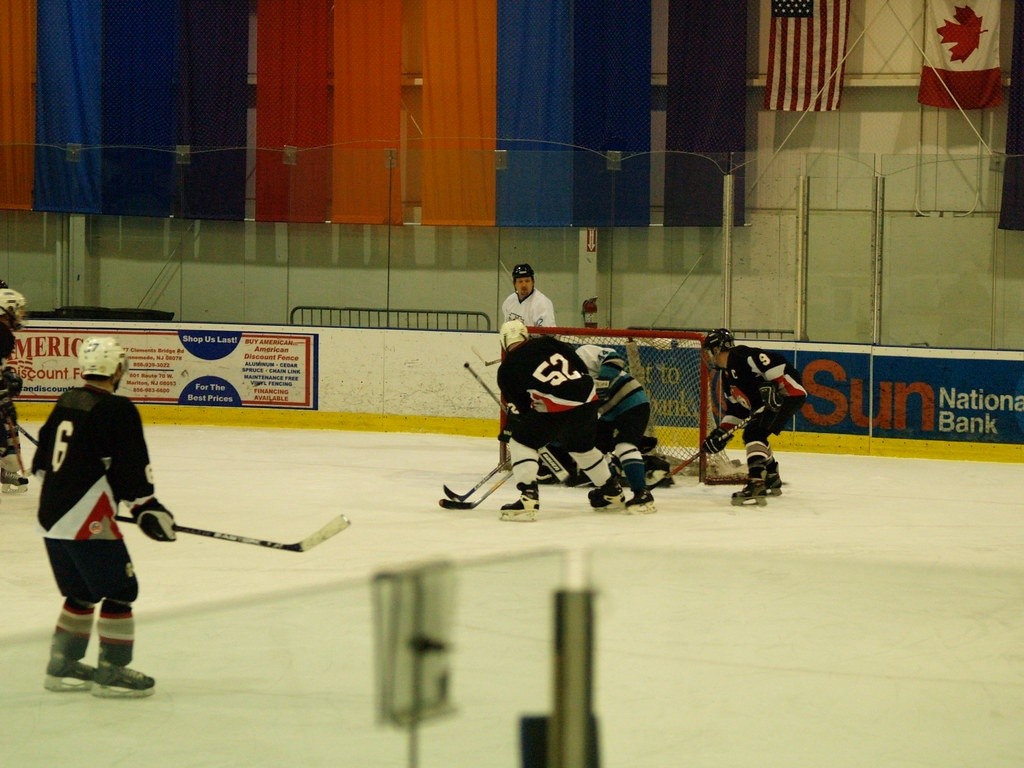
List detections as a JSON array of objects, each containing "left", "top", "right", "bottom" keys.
[{"left": 54, "top": 303, "right": 176, "bottom": 321}]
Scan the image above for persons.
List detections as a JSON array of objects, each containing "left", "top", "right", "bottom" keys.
[
  {"left": 0, "top": 282, "right": 26, "bottom": 486},
  {"left": 489, "top": 265, "right": 557, "bottom": 327},
  {"left": 699, "top": 327, "right": 811, "bottom": 500},
  {"left": 498, "top": 321, "right": 628, "bottom": 516},
  {"left": 572, "top": 345, "right": 654, "bottom": 506},
  {"left": 34, "top": 335, "right": 176, "bottom": 690}
]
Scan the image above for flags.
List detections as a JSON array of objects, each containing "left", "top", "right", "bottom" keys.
[
  {"left": 917, "top": 0, "right": 1003, "bottom": 110},
  {"left": 764, "top": 0, "right": 850, "bottom": 112}
]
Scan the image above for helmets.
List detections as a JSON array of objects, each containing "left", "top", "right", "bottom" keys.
[
  {"left": 702, "top": 329, "right": 735, "bottom": 353},
  {"left": 499, "top": 320, "right": 529, "bottom": 350},
  {"left": 512, "top": 263, "right": 534, "bottom": 279},
  {"left": 0, "top": 288, "right": 28, "bottom": 316},
  {"left": 78, "top": 337, "right": 127, "bottom": 380}
]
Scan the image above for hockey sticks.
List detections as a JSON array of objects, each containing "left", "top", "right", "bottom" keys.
[
  {"left": 7, "top": 415, "right": 33, "bottom": 477},
  {"left": 471, "top": 345, "right": 501, "bottom": 366},
  {"left": 463, "top": 361, "right": 571, "bottom": 485},
  {"left": 15, "top": 423, "right": 40, "bottom": 447},
  {"left": 438, "top": 472, "right": 514, "bottom": 511},
  {"left": 443, "top": 454, "right": 512, "bottom": 503},
  {"left": 643, "top": 404, "right": 767, "bottom": 491},
  {"left": 113, "top": 511, "right": 352, "bottom": 553}
]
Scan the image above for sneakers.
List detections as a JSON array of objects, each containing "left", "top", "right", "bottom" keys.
[
  {"left": 765, "top": 461, "right": 784, "bottom": 497},
  {"left": 588, "top": 477, "right": 626, "bottom": 513},
  {"left": 45, "top": 660, "right": 96, "bottom": 691},
  {"left": 92, "top": 663, "right": 155, "bottom": 699},
  {"left": 625, "top": 486, "right": 657, "bottom": 515},
  {"left": 500, "top": 480, "right": 539, "bottom": 522},
  {"left": 732, "top": 464, "right": 769, "bottom": 506},
  {"left": 1, "top": 468, "right": 28, "bottom": 494}
]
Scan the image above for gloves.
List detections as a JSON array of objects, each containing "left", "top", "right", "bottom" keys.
[
  {"left": 130, "top": 497, "right": 176, "bottom": 542},
  {"left": 702, "top": 427, "right": 729, "bottom": 453}
]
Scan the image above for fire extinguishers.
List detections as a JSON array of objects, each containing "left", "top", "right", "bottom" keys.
[{"left": 581, "top": 296, "right": 599, "bottom": 327}]
